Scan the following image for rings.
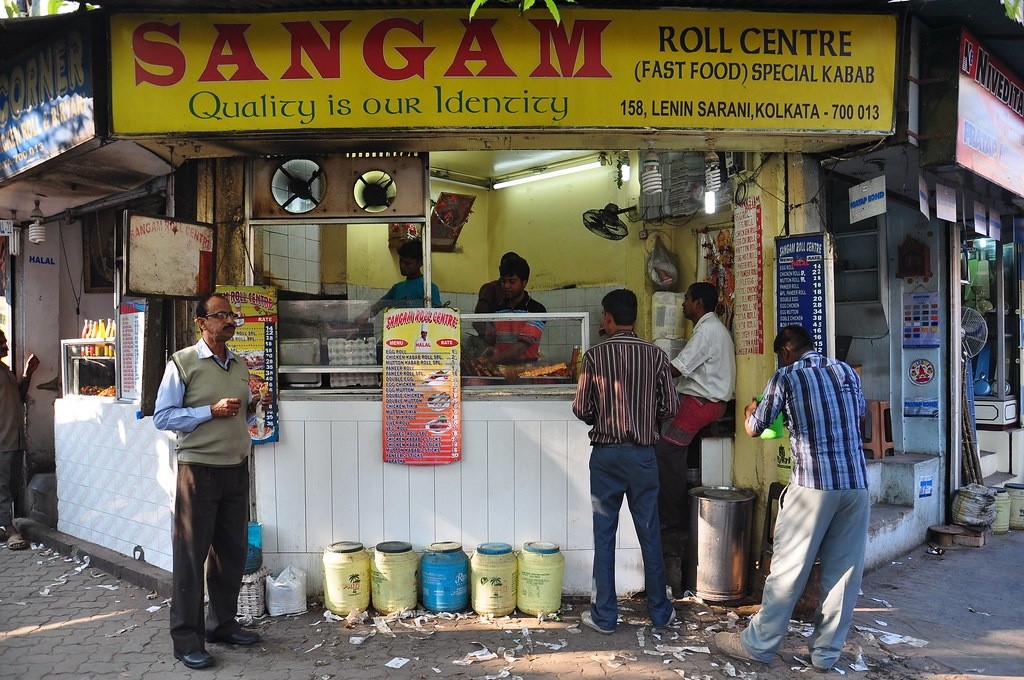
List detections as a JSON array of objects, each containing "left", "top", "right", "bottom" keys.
[{"left": 232, "top": 413, "right": 235, "bottom": 417}]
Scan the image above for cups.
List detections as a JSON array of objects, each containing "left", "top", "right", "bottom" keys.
[{"left": 757, "top": 394, "right": 784, "bottom": 439}]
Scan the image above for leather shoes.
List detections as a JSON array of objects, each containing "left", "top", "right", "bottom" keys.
[
  {"left": 206, "top": 627, "right": 259, "bottom": 644},
  {"left": 174, "top": 647, "right": 215, "bottom": 669}
]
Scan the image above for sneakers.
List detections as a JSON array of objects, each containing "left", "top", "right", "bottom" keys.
[
  {"left": 811, "top": 662, "right": 831, "bottom": 673},
  {"left": 715, "top": 630, "right": 764, "bottom": 665}
]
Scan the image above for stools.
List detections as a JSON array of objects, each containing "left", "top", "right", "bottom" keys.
[
  {"left": 859, "top": 400, "right": 894, "bottom": 460},
  {"left": 760, "top": 481, "right": 786, "bottom": 566}
]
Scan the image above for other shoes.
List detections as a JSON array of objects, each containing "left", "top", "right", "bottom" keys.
[
  {"left": 661, "top": 525, "right": 686, "bottom": 536},
  {"left": 581, "top": 611, "right": 615, "bottom": 635},
  {"left": 663, "top": 608, "right": 676, "bottom": 626}
]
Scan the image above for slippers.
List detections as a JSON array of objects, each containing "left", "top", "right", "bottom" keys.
[
  {"left": 6, "top": 533, "right": 26, "bottom": 549},
  {"left": 0, "top": 526, "right": 8, "bottom": 540}
]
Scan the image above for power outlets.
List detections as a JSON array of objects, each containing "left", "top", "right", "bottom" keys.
[{"left": 639, "top": 230, "right": 647, "bottom": 239}]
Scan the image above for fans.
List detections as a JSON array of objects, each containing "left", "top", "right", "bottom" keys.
[
  {"left": 959, "top": 306, "right": 988, "bottom": 361},
  {"left": 583, "top": 198, "right": 640, "bottom": 240}
]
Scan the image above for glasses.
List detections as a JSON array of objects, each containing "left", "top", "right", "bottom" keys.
[{"left": 204, "top": 311, "right": 235, "bottom": 319}]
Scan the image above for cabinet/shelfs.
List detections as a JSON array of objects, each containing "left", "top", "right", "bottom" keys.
[
  {"left": 829, "top": 199, "right": 890, "bottom": 336},
  {"left": 965, "top": 230, "right": 1020, "bottom": 431}
]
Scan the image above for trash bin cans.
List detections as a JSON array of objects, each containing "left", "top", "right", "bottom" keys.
[{"left": 687, "top": 484, "right": 758, "bottom": 600}]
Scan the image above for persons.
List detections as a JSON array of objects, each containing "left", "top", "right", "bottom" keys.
[
  {"left": 0, "top": 329, "right": 40, "bottom": 550},
  {"left": 479, "top": 256, "right": 547, "bottom": 365},
  {"left": 715, "top": 324, "right": 871, "bottom": 672},
  {"left": 572, "top": 289, "right": 677, "bottom": 634},
  {"left": 652, "top": 282, "right": 736, "bottom": 537},
  {"left": 153, "top": 294, "right": 274, "bottom": 669},
  {"left": 471, "top": 251, "right": 519, "bottom": 336},
  {"left": 354, "top": 240, "right": 442, "bottom": 324}
]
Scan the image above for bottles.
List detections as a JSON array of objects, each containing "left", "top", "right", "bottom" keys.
[
  {"left": 79, "top": 317, "right": 116, "bottom": 357},
  {"left": 571, "top": 344, "right": 583, "bottom": 383}
]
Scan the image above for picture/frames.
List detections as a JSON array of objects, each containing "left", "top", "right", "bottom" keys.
[
  {"left": 82, "top": 193, "right": 167, "bottom": 293},
  {"left": 430, "top": 192, "right": 476, "bottom": 252}
]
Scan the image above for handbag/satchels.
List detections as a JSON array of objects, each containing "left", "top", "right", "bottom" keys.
[
  {"left": 647, "top": 235, "right": 678, "bottom": 289},
  {"left": 265, "top": 566, "right": 307, "bottom": 614}
]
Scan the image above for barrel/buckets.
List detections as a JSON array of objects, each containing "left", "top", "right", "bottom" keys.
[
  {"left": 517, "top": 541, "right": 565, "bottom": 618},
  {"left": 372, "top": 541, "right": 420, "bottom": 615},
  {"left": 243, "top": 522, "right": 262, "bottom": 575},
  {"left": 1003, "top": 483, "right": 1024, "bottom": 529},
  {"left": 681, "top": 485, "right": 756, "bottom": 601},
  {"left": 469, "top": 544, "right": 517, "bottom": 617},
  {"left": 322, "top": 541, "right": 372, "bottom": 616},
  {"left": 421, "top": 541, "right": 469, "bottom": 614},
  {"left": 987, "top": 486, "right": 1011, "bottom": 535}
]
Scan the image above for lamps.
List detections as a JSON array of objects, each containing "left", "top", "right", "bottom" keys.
[
  {"left": 490, "top": 151, "right": 609, "bottom": 190},
  {"left": 430, "top": 166, "right": 491, "bottom": 190}
]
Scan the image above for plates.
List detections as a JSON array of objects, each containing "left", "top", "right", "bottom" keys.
[
  {"left": 247, "top": 421, "right": 275, "bottom": 440},
  {"left": 974, "top": 380, "right": 1011, "bottom": 396},
  {"left": 424, "top": 371, "right": 452, "bottom": 433}
]
